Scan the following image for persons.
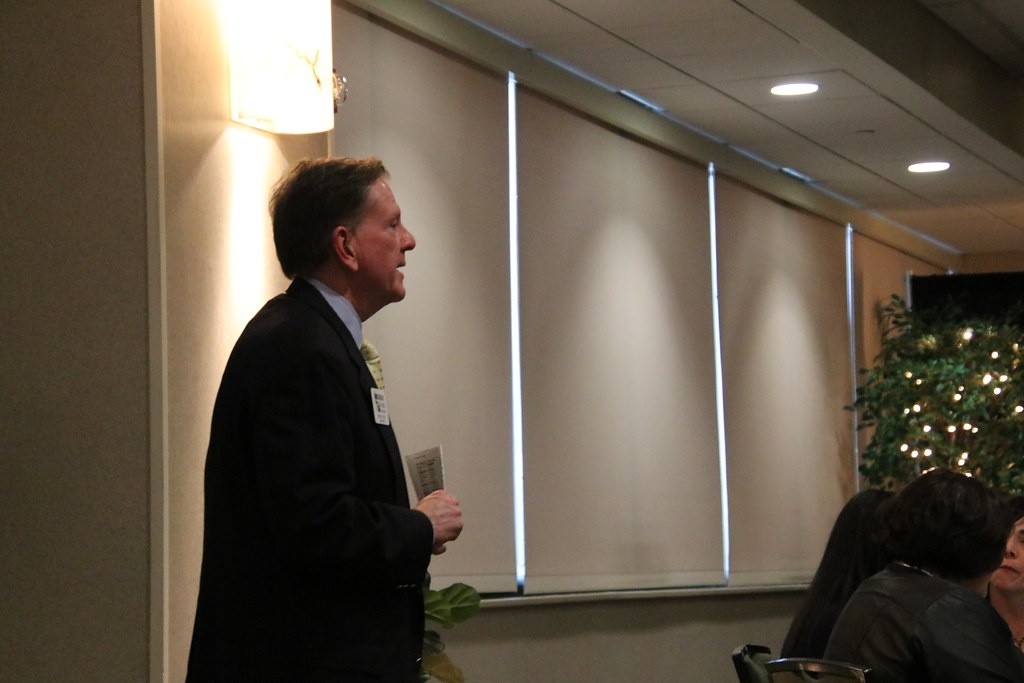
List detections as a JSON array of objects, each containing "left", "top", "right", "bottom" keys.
[
  {"left": 185, "top": 159, "right": 463, "bottom": 683},
  {"left": 780, "top": 465, "right": 1024, "bottom": 683}
]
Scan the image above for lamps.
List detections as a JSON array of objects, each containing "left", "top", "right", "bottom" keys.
[{"left": 230, "top": 0, "right": 336, "bottom": 137}]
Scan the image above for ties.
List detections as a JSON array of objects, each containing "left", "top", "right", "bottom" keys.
[{"left": 360, "top": 341, "right": 386, "bottom": 404}]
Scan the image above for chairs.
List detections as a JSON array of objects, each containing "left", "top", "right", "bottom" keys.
[
  {"left": 764, "top": 657, "right": 873, "bottom": 683},
  {"left": 731, "top": 642, "right": 778, "bottom": 683}
]
